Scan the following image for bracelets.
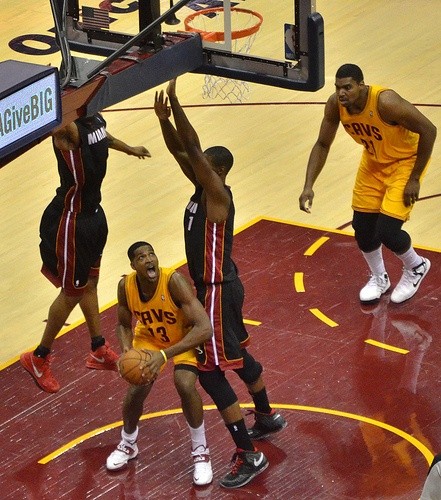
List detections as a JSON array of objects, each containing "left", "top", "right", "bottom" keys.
[{"left": 160, "top": 349, "right": 168, "bottom": 365}]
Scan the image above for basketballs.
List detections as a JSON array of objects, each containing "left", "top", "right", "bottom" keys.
[{"left": 117, "top": 347, "right": 158, "bottom": 386}]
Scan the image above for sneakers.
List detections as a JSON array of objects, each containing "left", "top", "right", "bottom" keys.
[
  {"left": 20, "top": 350, "right": 61, "bottom": 393},
  {"left": 85, "top": 342, "right": 120, "bottom": 371},
  {"left": 247, "top": 408, "right": 288, "bottom": 441},
  {"left": 106, "top": 439, "right": 139, "bottom": 471},
  {"left": 191, "top": 444, "right": 212, "bottom": 486},
  {"left": 359, "top": 272, "right": 391, "bottom": 305},
  {"left": 391, "top": 256, "right": 431, "bottom": 303},
  {"left": 219, "top": 450, "right": 269, "bottom": 489}
]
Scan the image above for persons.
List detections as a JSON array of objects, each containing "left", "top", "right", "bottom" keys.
[
  {"left": 154, "top": 74, "right": 288, "bottom": 490},
  {"left": 106, "top": 241, "right": 215, "bottom": 487},
  {"left": 22, "top": 111, "right": 152, "bottom": 396},
  {"left": 298, "top": 64, "right": 438, "bottom": 304}
]
